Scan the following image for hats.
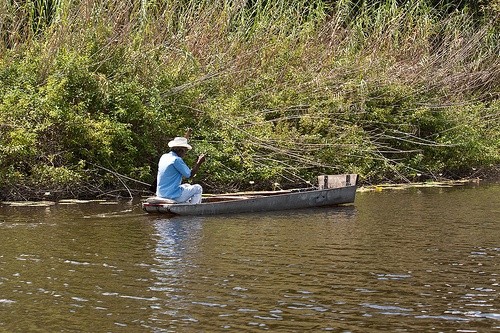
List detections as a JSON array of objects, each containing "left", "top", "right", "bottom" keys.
[{"left": 168, "top": 136, "right": 192, "bottom": 150}]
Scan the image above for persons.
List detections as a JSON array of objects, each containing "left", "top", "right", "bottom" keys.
[{"left": 156, "top": 136, "right": 205, "bottom": 203}]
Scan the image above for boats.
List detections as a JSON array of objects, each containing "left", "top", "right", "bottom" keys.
[{"left": 141, "top": 173, "right": 360, "bottom": 216}]
